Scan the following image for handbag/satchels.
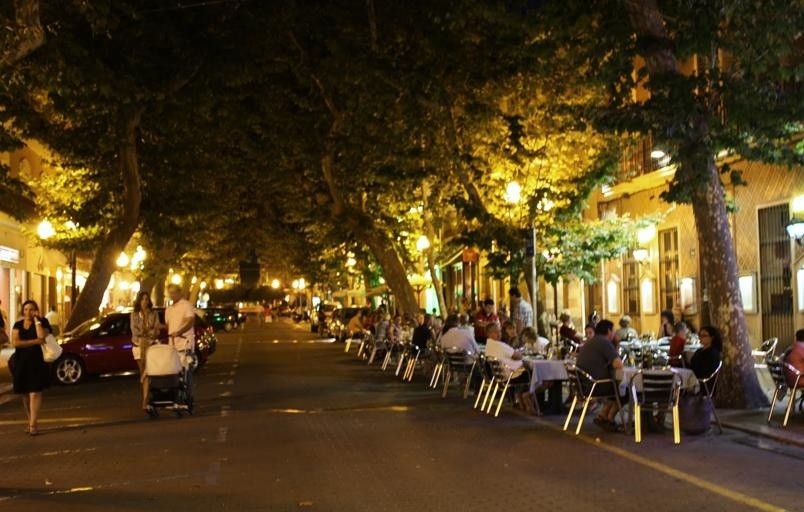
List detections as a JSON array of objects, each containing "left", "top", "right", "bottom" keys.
[{"left": 41, "top": 334, "right": 63, "bottom": 363}]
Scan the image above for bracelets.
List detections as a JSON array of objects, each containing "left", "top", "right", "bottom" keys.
[{"left": 34, "top": 321, "right": 42, "bottom": 326}]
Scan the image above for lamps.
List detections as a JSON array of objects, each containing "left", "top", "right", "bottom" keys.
[
  {"left": 782, "top": 212, "right": 804, "bottom": 246},
  {"left": 650, "top": 141, "right": 667, "bottom": 161},
  {"left": 630, "top": 246, "right": 650, "bottom": 271}
]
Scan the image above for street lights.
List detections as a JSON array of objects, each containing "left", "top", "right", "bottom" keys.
[{"left": 38, "top": 221, "right": 79, "bottom": 313}]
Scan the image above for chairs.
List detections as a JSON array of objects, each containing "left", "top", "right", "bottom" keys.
[{"left": 343, "top": 321, "right": 804, "bottom": 445}]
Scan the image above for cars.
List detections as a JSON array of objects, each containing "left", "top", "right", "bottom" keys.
[
  {"left": 7, "top": 305, "right": 246, "bottom": 385},
  {"left": 279, "top": 299, "right": 370, "bottom": 342}
]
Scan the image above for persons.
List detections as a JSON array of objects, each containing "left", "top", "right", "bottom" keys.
[
  {"left": 658, "top": 310, "right": 675, "bottom": 339},
  {"left": 131, "top": 291, "right": 161, "bottom": 411},
  {"left": 502, "top": 321, "right": 519, "bottom": 349},
  {"left": 671, "top": 321, "right": 688, "bottom": 367},
  {"left": 580, "top": 319, "right": 629, "bottom": 432},
  {"left": 522, "top": 326, "right": 551, "bottom": 357},
  {"left": 585, "top": 313, "right": 600, "bottom": 340},
  {"left": 11, "top": 299, "right": 52, "bottom": 436},
  {"left": 616, "top": 315, "right": 637, "bottom": 341},
  {"left": 560, "top": 313, "right": 583, "bottom": 344},
  {"left": 347, "top": 298, "right": 499, "bottom": 394},
  {"left": 509, "top": 288, "right": 533, "bottom": 331},
  {"left": 679, "top": 325, "right": 723, "bottom": 434},
  {"left": 0, "top": 300, "right": 8, "bottom": 354},
  {"left": 165, "top": 284, "right": 195, "bottom": 403},
  {"left": 486, "top": 323, "right": 531, "bottom": 411},
  {"left": 784, "top": 329, "right": 804, "bottom": 389},
  {"left": 318, "top": 307, "right": 326, "bottom": 336}
]
